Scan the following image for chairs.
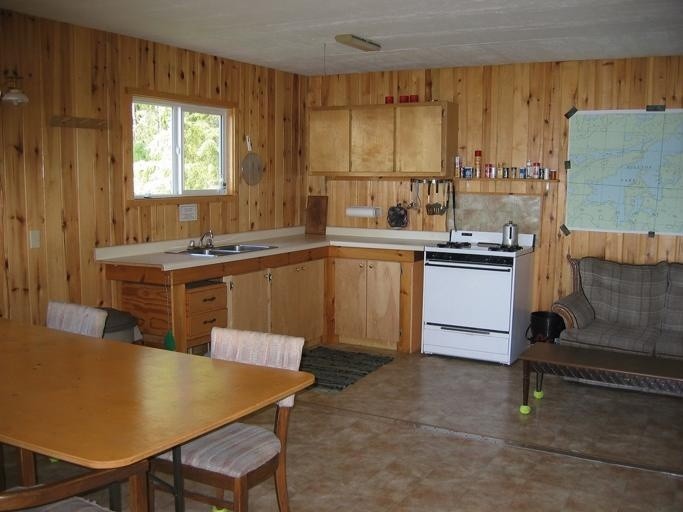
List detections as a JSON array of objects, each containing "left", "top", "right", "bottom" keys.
[
  {"left": 149, "top": 322, "right": 308, "bottom": 512},
  {"left": 13, "top": 297, "right": 112, "bottom": 485},
  {"left": 2, "top": 482, "right": 111, "bottom": 512}
]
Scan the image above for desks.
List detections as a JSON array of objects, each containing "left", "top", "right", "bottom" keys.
[{"left": 0, "top": 316, "right": 316, "bottom": 510}]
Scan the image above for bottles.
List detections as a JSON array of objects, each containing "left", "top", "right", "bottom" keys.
[{"left": 453, "top": 149, "right": 557, "bottom": 180}]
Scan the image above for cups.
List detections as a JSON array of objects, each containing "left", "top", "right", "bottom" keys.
[
  {"left": 399, "top": 95, "right": 409, "bottom": 103},
  {"left": 409, "top": 95, "right": 419, "bottom": 103},
  {"left": 385, "top": 96, "right": 394, "bottom": 104}
]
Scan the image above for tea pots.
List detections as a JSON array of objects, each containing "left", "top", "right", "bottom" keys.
[{"left": 500, "top": 220, "right": 519, "bottom": 248}]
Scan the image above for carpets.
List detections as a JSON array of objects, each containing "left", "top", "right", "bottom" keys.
[{"left": 285, "top": 341, "right": 396, "bottom": 394}]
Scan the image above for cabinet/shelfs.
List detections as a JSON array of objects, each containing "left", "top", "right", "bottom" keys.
[
  {"left": 225, "top": 251, "right": 270, "bottom": 334},
  {"left": 302, "top": 99, "right": 460, "bottom": 181},
  {"left": 331, "top": 243, "right": 423, "bottom": 353},
  {"left": 267, "top": 244, "right": 329, "bottom": 354},
  {"left": 106, "top": 262, "right": 226, "bottom": 358}
]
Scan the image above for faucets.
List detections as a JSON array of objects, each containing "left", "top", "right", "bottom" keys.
[{"left": 199, "top": 229, "right": 213, "bottom": 249}]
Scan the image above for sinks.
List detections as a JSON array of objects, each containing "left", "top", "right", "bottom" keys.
[
  {"left": 187, "top": 250, "right": 239, "bottom": 257},
  {"left": 212, "top": 243, "right": 277, "bottom": 252}
]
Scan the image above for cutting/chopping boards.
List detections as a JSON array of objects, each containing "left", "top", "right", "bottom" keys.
[{"left": 304, "top": 195, "right": 327, "bottom": 235}]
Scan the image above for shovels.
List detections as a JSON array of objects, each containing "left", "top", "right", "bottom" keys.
[{"left": 426, "top": 180, "right": 440, "bottom": 215}]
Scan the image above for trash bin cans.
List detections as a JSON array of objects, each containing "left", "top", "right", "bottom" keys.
[
  {"left": 94, "top": 305, "right": 138, "bottom": 343},
  {"left": 524, "top": 312, "right": 561, "bottom": 343}
]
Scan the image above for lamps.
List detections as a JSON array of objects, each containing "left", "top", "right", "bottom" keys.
[
  {"left": 334, "top": 32, "right": 383, "bottom": 52},
  {"left": 0, "top": 69, "right": 31, "bottom": 111}
]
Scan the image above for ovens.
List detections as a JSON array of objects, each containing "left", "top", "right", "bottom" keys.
[{"left": 420, "top": 261, "right": 533, "bottom": 367}]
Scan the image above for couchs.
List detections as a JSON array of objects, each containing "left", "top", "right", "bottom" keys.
[{"left": 547, "top": 250, "right": 682, "bottom": 402}]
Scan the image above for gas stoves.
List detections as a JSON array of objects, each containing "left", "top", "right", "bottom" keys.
[{"left": 424, "top": 242, "right": 533, "bottom": 266}]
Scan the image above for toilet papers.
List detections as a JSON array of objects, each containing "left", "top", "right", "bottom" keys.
[{"left": 345, "top": 206, "right": 380, "bottom": 218}]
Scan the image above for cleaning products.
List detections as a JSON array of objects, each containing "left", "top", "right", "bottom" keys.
[{"left": 455, "top": 149, "right": 557, "bottom": 180}]
